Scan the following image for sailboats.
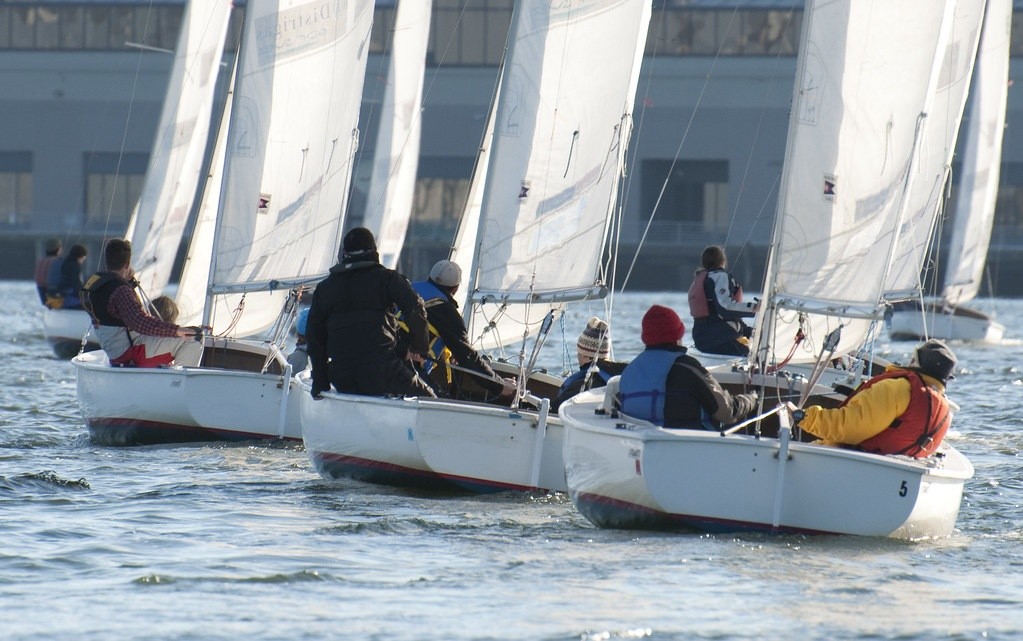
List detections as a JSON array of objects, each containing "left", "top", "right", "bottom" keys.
[
  {"left": 557, "top": 0, "right": 1015, "bottom": 541},
  {"left": 888, "top": 0, "right": 1008, "bottom": 347},
  {"left": 291, "top": 0, "right": 653, "bottom": 496},
  {"left": 45, "top": 1, "right": 433, "bottom": 445}
]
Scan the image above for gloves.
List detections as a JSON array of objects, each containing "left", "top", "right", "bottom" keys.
[{"left": 750, "top": 389, "right": 760, "bottom": 407}]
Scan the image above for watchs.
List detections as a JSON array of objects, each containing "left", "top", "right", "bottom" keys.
[{"left": 792, "top": 407, "right": 804, "bottom": 421}]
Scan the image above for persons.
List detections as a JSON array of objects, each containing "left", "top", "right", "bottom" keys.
[
  {"left": 779, "top": 339, "right": 958, "bottom": 460},
  {"left": 549, "top": 316, "right": 627, "bottom": 415},
  {"left": 85, "top": 239, "right": 205, "bottom": 369},
  {"left": 286, "top": 227, "right": 518, "bottom": 401},
  {"left": 617, "top": 305, "right": 757, "bottom": 433},
  {"left": 34, "top": 237, "right": 88, "bottom": 308},
  {"left": 688, "top": 247, "right": 762, "bottom": 363}
]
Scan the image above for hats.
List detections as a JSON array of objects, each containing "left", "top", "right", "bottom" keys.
[
  {"left": 641, "top": 305, "right": 686, "bottom": 345},
  {"left": 343, "top": 228, "right": 377, "bottom": 256},
  {"left": 46, "top": 238, "right": 63, "bottom": 252},
  {"left": 913, "top": 338, "right": 958, "bottom": 382},
  {"left": 576, "top": 317, "right": 610, "bottom": 358},
  {"left": 429, "top": 260, "right": 462, "bottom": 287},
  {"left": 297, "top": 308, "right": 310, "bottom": 336}
]
